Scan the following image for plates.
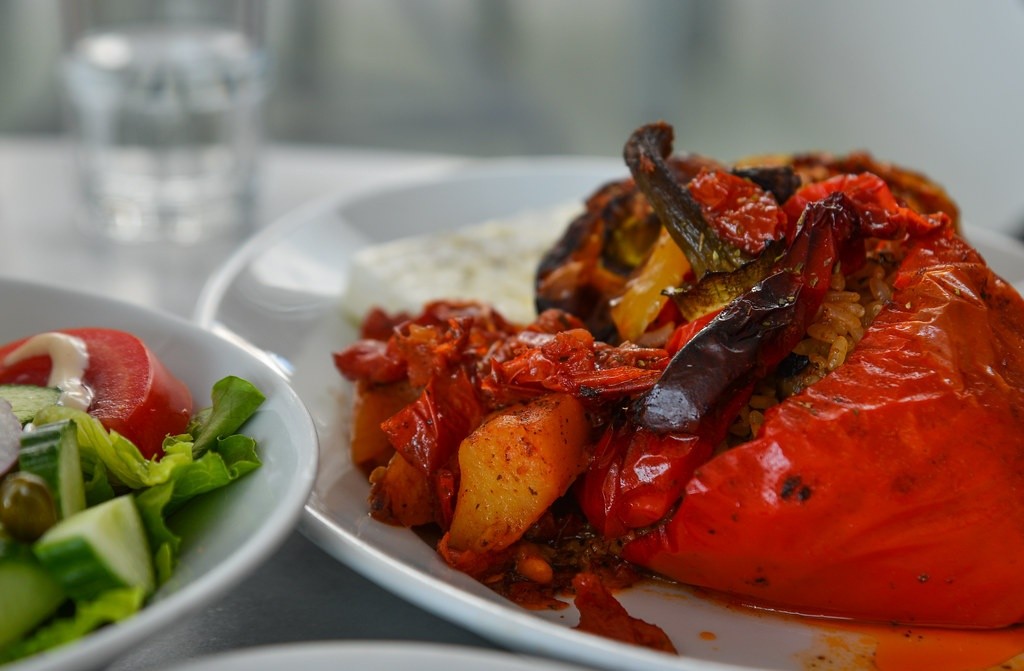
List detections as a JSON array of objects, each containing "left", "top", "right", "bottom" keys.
[
  {"left": 3, "top": 274, "right": 320, "bottom": 657},
  {"left": 202, "top": 157, "right": 1023, "bottom": 668}
]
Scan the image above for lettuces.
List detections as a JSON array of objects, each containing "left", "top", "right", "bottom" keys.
[{"left": 1, "top": 375, "right": 264, "bottom": 667}]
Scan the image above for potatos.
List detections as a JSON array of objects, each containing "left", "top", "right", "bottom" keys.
[{"left": 447, "top": 392, "right": 588, "bottom": 555}]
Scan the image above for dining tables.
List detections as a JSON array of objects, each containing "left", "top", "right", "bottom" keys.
[{"left": 0, "top": 134, "right": 494, "bottom": 671}]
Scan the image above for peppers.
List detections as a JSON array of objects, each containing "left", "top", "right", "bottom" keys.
[{"left": 582, "top": 116, "right": 1021, "bottom": 633}]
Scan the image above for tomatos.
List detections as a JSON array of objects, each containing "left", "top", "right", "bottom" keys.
[{"left": 1, "top": 330, "right": 196, "bottom": 457}]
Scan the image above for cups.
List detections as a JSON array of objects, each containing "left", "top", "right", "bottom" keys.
[{"left": 54, "top": 0, "right": 275, "bottom": 235}]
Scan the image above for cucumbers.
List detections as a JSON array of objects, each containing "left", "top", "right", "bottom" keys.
[{"left": 2, "top": 419, "right": 154, "bottom": 650}]
